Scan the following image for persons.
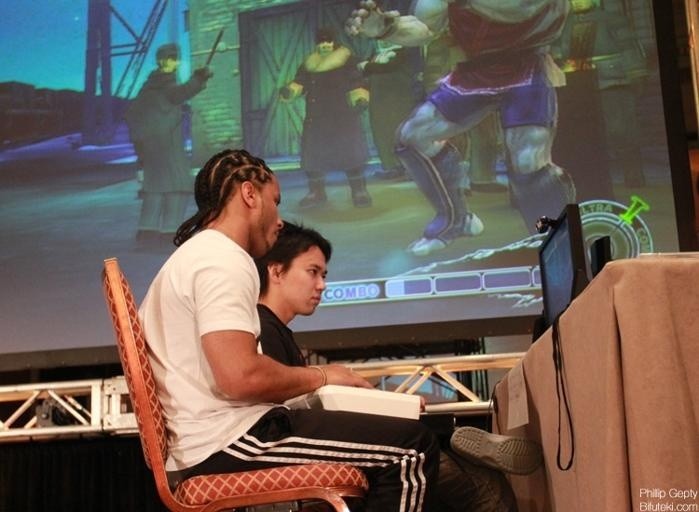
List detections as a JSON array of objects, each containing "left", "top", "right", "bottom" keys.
[
  {"left": 128, "top": 42, "right": 214, "bottom": 250},
  {"left": 137, "top": 148, "right": 441, "bottom": 512},
  {"left": 252, "top": 219, "right": 544, "bottom": 512},
  {"left": 280, "top": 0, "right": 671, "bottom": 257}
]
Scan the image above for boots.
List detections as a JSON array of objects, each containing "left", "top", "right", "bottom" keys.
[
  {"left": 349, "top": 175, "right": 373, "bottom": 208},
  {"left": 301, "top": 176, "right": 327, "bottom": 208}
]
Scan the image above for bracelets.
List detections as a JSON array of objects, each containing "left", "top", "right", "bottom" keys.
[{"left": 308, "top": 366, "right": 326, "bottom": 386}]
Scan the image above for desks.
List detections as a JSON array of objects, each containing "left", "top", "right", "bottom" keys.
[{"left": 486, "top": 253, "right": 699, "bottom": 511}]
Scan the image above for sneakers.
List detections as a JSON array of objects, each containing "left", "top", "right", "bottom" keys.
[
  {"left": 450, "top": 427, "right": 544, "bottom": 475},
  {"left": 415, "top": 211, "right": 485, "bottom": 256},
  {"left": 162, "top": 229, "right": 184, "bottom": 243},
  {"left": 137, "top": 228, "right": 159, "bottom": 248}
]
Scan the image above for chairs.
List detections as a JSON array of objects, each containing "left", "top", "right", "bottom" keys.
[{"left": 101, "top": 257, "right": 371, "bottom": 512}]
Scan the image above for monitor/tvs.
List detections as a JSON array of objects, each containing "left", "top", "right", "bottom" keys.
[{"left": 534, "top": 202, "right": 591, "bottom": 327}]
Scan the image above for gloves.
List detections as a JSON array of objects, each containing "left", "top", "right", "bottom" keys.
[
  {"left": 353, "top": 95, "right": 369, "bottom": 112},
  {"left": 195, "top": 65, "right": 210, "bottom": 80},
  {"left": 278, "top": 83, "right": 291, "bottom": 99}
]
